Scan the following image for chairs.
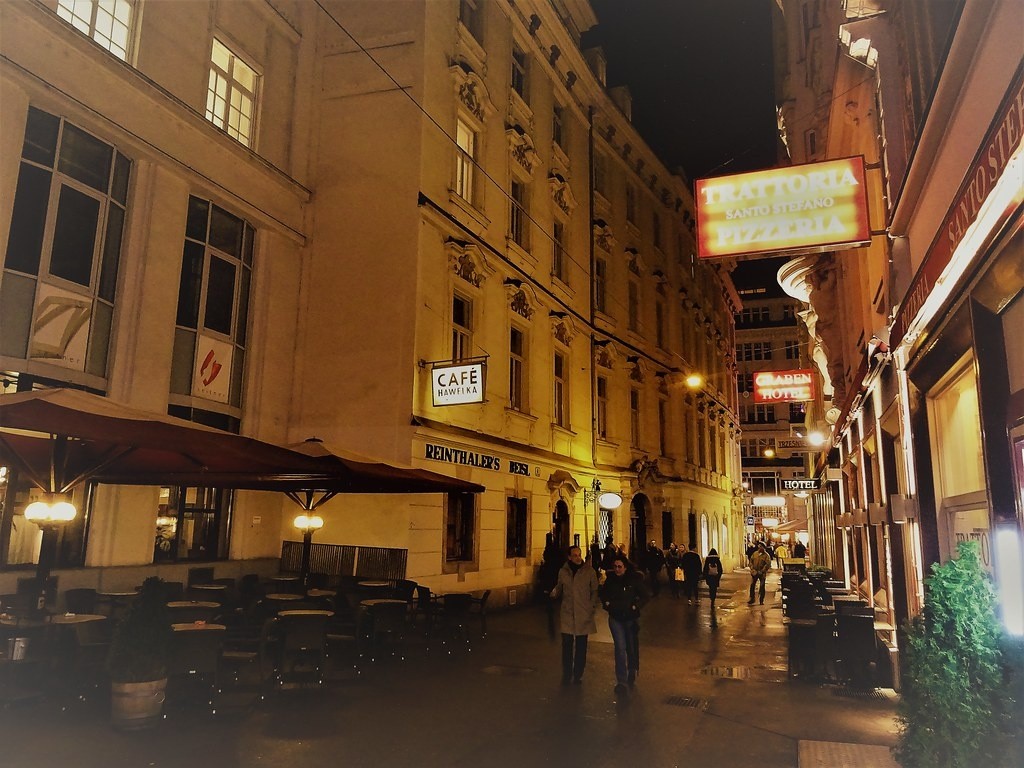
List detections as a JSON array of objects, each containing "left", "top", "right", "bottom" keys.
[
  {"left": 461, "top": 589, "right": 492, "bottom": 639},
  {"left": 837, "top": 614, "right": 878, "bottom": 679},
  {"left": 789, "top": 614, "right": 837, "bottom": 681},
  {"left": 435, "top": 594, "right": 474, "bottom": 651},
  {"left": 281, "top": 612, "right": 328, "bottom": 692},
  {"left": 308, "top": 572, "right": 329, "bottom": 591},
  {"left": 782, "top": 571, "right": 823, "bottom": 616},
  {"left": 175, "top": 631, "right": 218, "bottom": 695},
  {"left": 240, "top": 575, "right": 260, "bottom": 603},
  {"left": 218, "top": 616, "right": 277, "bottom": 694},
  {"left": 326, "top": 605, "right": 372, "bottom": 663},
  {"left": 368, "top": 601, "right": 406, "bottom": 661},
  {"left": 840, "top": 605, "right": 875, "bottom": 620},
  {"left": 411, "top": 586, "right": 443, "bottom": 639},
  {"left": 833, "top": 599, "right": 866, "bottom": 615},
  {"left": 0, "top": 566, "right": 129, "bottom": 707}
]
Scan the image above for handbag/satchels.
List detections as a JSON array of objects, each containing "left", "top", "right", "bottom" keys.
[{"left": 675, "top": 567, "right": 684, "bottom": 581}]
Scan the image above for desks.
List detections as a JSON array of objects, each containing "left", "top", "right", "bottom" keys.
[
  {"left": 271, "top": 575, "right": 300, "bottom": 595},
  {"left": 95, "top": 592, "right": 138, "bottom": 623},
  {"left": 191, "top": 584, "right": 227, "bottom": 600},
  {"left": 278, "top": 610, "right": 335, "bottom": 618},
  {"left": 783, "top": 605, "right": 886, "bottom": 617},
  {"left": 166, "top": 600, "right": 223, "bottom": 622},
  {"left": 783, "top": 618, "right": 894, "bottom": 678},
  {"left": 361, "top": 598, "right": 408, "bottom": 651},
  {"left": 825, "top": 587, "right": 852, "bottom": 595},
  {"left": 358, "top": 581, "right": 389, "bottom": 599},
  {"left": 172, "top": 623, "right": 225, "bottom": 677},
  {"left": 307, "top": 590, "right": 337, "bottom": 611},
  {"left": 825, "top": 580, "right": 843, "bottom": 585},
  {"left": 43, "top": 614, "right": 107, "bottom": 647},
  {"left": 267, "top": 594, "right": 304, "bottom": 616}
]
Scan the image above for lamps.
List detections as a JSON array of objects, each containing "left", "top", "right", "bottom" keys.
[
  {"left": 891, "top": 494, "right": 914, "bottom": 525},
  {"left": 853, "top": 508, "right": 867, "bottom": 528},
  {"left": 836, "top": 515, "right": 845, "bottom": 529},
  {"left": 309, "top": 516, "right": 324, "bottom": 534},
  {"left": 24, "top": 499, "right": 48, "bottom": 530},
  {"left": 50, "top": 502, "right": 77, "bottom": 530},
  {"left": 869, "top": 502, "right": 886, "bottom": 526},
  {"left": 844, "top": 512, "right": 855, "bottom": 530},
  {"left": 294, "top": 516, "right": 308, "bottom": 535}
]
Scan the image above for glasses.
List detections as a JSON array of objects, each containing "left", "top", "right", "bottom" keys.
[{"left": 614, "top": 564, "right": 623, "bottom": 568}]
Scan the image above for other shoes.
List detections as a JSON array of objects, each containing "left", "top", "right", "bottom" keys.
[
  {"left": 574, "top": 681, "right": 582, "bottom": 686},
  {"left": 695, "top": 601, "right": 699, "bottom": 605},
  {"left": 759, "top": 601, "right": 764, "bottom": 605},
  {"left": 614, "top": 684, "right": 626, "bottom": 693},
  {"left": 688, "top": 600, "right": 692, "bottom": 606},
  {"left": 561, "top": 678, "right": 571, "bottom": 687},
  {"left": 747, "top": 599, "right": 754, "bottom": 604}
]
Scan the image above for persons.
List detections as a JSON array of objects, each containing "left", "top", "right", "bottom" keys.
[
  {"left": 748, "top": 539, "right": 807, "bottom": 570},
  {"left": 540, "top": 542, "right": 564, "bottom": 636},
  {"left": 643, "top": 540, "right": 665, "bottom": 588},
  {"left": 681, "top": 542, "right": 703, "bottom": 606},
  {"left": 585, "top": 537, "right": 628, "bottom": 597},
  {"left": 665, "top": 542, "right": 681, "bottom": 598},
  {"left": 703, "top": 548, "right": 723, "bottom": 611},
  {"left": 678, "top": 543, "right": 687, "bottom": 557},
  {"left": 748, "top": 541, "right": 771, "bottom": 605},
  {"left": 601, "top": 554, "right": 650, "bottom": 698},
  {"left": 548, "top": 545, "right": 600, "bottom": 686}
]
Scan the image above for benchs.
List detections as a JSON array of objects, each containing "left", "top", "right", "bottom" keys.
[{"left": 341, "top": 575, "right": 418, "bottom": 623}]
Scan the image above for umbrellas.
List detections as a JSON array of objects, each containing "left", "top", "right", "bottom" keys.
[
  {"left": 1, "top": 388, "right": 343, "bottom": 626},
  {"left": 279, "top": 433, "right": 485, "bottom": 596}
]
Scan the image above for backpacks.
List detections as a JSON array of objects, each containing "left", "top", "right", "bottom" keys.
[{"left": 708, "top": 563, "right": 718, "bottom": 575}]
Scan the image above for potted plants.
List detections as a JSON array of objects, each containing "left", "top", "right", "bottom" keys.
[{"left": 105, "top": 576, "right": 172, "bottom": 735}]
